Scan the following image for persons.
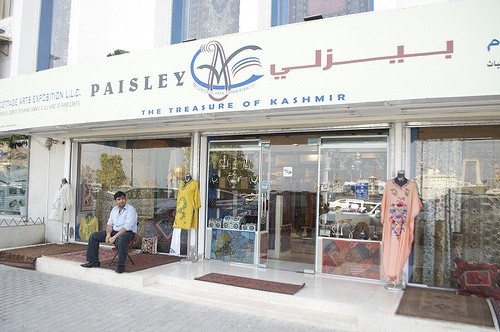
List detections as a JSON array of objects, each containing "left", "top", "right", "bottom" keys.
[
  {"left": 78, "top": 212, "right": 99, "bottom": 242},
  {"left": 173, "top": 173, "right": 202, "bottom": 229},
  {"left": 81, "top": 190, "right": 138, "bottom": 274},
  {"left": 48, "top": 177, "right": 73, "bottom": 223},
  {"left": 380, "top": 169, "right": 423, "bottom": 290}
]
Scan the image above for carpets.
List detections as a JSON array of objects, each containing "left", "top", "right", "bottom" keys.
[
  {"left": 194, "top": 272, "right": 305, "bottom": 295},
  {"left": 0, "top": 242, "right": 187, "bottom": 274},
  {"left": 395, "top": 285, "right": 495, "bottom": 327}
]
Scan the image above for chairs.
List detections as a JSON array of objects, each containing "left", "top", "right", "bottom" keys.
[{"left": 109, "top": 222, "right": 140, "bottom": 266}]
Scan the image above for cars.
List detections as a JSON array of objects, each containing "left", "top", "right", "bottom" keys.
[{"left": 320, "top": 198, "right": 382, "bottom": 240}]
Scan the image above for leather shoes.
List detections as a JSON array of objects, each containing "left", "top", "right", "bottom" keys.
[
  {"left": 115, "top": 265, "right": 125, "bottom": 273},
  {"left": 80, "top": 262, "right": 100, "bottom": 267}
]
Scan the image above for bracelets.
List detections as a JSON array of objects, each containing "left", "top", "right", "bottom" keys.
[{"left": 113, "top": 235, "right": 116, "bottom": 239}]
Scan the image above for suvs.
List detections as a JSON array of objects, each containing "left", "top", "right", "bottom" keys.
[{"left": 81, "top": 183, "right": 179, "bottom": 224}]
